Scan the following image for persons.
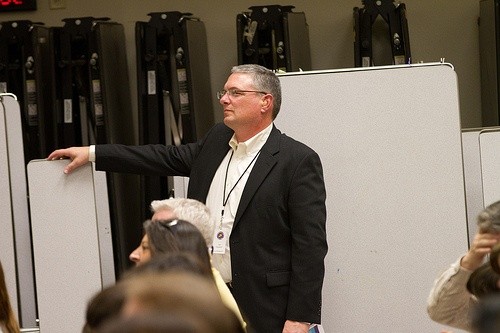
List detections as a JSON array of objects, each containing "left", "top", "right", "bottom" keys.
[
  {"left": 428, "top": 200, "right": 500, "bottom": 333},
  {"left": 1, "top": 196, "right": 245, "bottom": 332},
  {"left": 45, "top": 64, "right": 329, "bottom": 333}
]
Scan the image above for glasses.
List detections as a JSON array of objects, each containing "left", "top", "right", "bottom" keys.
[{"left": 217, "top": 89, "right": 268, "bottom": 100}]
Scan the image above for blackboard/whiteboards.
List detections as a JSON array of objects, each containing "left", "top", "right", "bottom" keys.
[
  {"left": 231, "top": 61, "right": 499, "bottom": 333},
  {"left": 0, "top": 89, "right": 119, "bottom": 333}
]
